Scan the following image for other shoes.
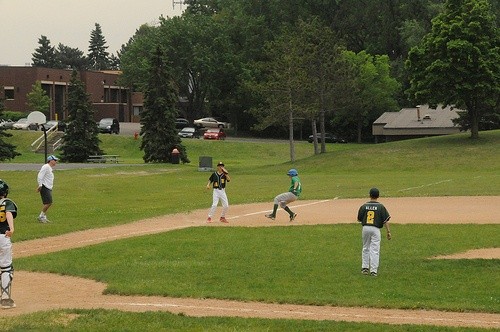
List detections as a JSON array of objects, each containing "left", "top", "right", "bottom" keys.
[
  {"left": 45, "top": 216, "right": 50, "bottom": 222},
  {"left": 37, "top": 216, "right": 47, "bottom": 224}
]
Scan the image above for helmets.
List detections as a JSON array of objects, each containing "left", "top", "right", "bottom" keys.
[
  {"left": 286, "top": 169, "right": 298, "bottom": 176},
  {"left": 0, "top": 179, "right": 9, "bottom": 196}
]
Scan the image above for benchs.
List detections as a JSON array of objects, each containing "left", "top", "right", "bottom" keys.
[{"left": 86, "top": 159, "right": 123, "bottom": 163}]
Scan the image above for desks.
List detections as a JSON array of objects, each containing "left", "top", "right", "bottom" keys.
[{"left": 89, "top": 155, "right": 121, "bottom": 165}]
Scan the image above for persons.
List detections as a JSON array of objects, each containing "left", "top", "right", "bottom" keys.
[
  {"left": 0, "top": 179, "right": 18, "bottom": 309},
  {"left": 357, "top": 188, "right": 391, "bottom": 276},
  {"left": 36, "top": 155, "right": 60, "bottom": 223},
  {"left": 265, "top": 169, "right": 303, "bottom": 223},
  {"left": 206, "top": 162, "right": 230, "bottom": 223}
]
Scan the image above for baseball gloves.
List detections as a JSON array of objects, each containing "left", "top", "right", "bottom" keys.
[{"left": 222, "top": 168, "right": 228, "bottom": 174}]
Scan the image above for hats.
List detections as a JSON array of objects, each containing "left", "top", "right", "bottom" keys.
[
  {"left": 370, "top": 188, "right": 379, "bottom": 198},
  {"left": 217, "top": 162, "right": 224, "bottom": 166},
  {"left": 46, "top": 155, "right": 58, "bottom": 163}
]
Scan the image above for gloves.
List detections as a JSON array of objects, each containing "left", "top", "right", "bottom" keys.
[{"left": 38, "top": 186, "right": 42, "bottom": 192}]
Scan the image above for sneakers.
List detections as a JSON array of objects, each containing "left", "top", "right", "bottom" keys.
[
  {"left": 361, "top": 268, "right": 368, "bottom": 274},
  {"left": 1, "top": 299, "right": 17, "bottom": 308},
  {"left": 219, "top": 217, "right": 229, "bottom": 223},
  {"left": 265, "top": 214, "right": 276, "bottom": 220},
  {"left": 370, "top": 272, "right": 377, "bottom": 276},
  {"left": 207, "top": 218, "right": 212, "bottom": 223},
  {"left": 289, "top": 213, "right": 297, "bottom": 222}
]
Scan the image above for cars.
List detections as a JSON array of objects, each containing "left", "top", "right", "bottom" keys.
[
  {"left": 41, "top": 120, "right": 65, "bottom": 131},
  {"left": 179, "top": 127, "right": 200, "bottom": 138},
  {"left": 0, "top": 118, "right": 14, "bottom": 130},
  {"left": 12, "top": 118, "right": 40, "bottom": 131},
  {"left": 204, "top": 128, "right": 226, "bottom": 140},
  {"left": 308, "top": 132, "right": 339, "bottom": 143},
  {"left": 193, "top": 118, "right": 226, "bottom": 128}
]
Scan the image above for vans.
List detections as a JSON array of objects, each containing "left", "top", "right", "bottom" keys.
[{"left": 97, "top": 118, "right": 120, "bottom": 134}]
